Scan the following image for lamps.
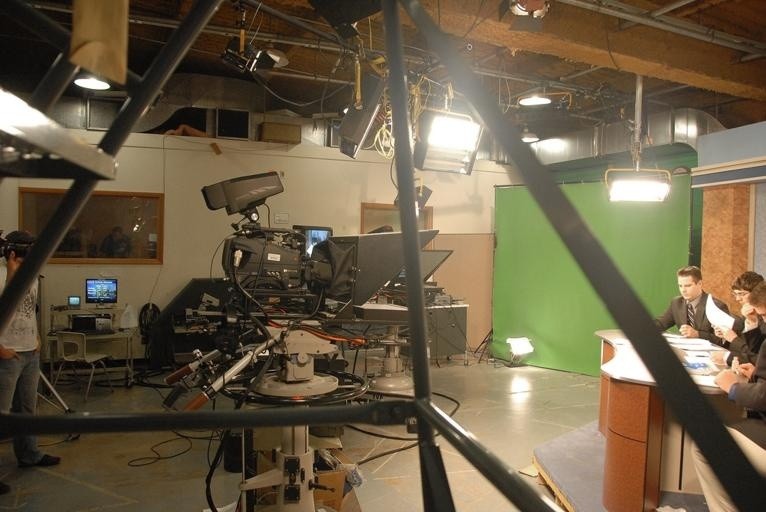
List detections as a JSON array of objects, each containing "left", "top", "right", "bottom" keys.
[
  {"left": 506, "top": 336, "right": 533, "bottom": 366},
  {"left": 71, "top": 68, "right": 114, "bottom": 93},
  {"left": 222, "top": 7, "right": 291, "bottom": 76},
  {"left": 606, "top": 70, "right": 673, "bottom": 204},
  {"left": 342, "top": 74, "right": 566, "bottom": 180}
]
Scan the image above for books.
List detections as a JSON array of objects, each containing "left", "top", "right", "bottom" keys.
[{"left": 662, "top": 332, "right": 723, "bottom": 388}]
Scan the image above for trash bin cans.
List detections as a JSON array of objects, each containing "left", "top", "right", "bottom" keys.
[{"left": 224, "top": 428, "right": 253, "bottom": 473}]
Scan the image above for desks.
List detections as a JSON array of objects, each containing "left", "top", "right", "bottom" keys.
[
  {"left": 43, "top": 304, "right": 139, "bottom": 388},
  {"left": 593, "top": 327, "right": 751, "bottom": 510}
]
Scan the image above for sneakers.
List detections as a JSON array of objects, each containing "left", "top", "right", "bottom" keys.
[
  {"left": 19, "top": 455, "right": 60, "bottom": 468},
  {"left": 0, "top": 482, "right": 10, "bottom": 495}
]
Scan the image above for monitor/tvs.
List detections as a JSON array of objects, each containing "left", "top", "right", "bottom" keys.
[
  {"left": 82, "top": 276, "right": 120, "bottom": 308},
  {"left": 68, "top": 296, "right": 80, "bottom": 306},
  {"left": 292, "top": 224, "right": 333, "bottom": 259}
]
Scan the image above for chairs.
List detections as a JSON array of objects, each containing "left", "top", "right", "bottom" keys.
[{"left": 47, "top": 329, "right": 115, "bottom": 402}]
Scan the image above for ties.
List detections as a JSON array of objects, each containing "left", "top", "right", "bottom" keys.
[{"left": 687, "top": 304, "right": 695, "bottom": 326}]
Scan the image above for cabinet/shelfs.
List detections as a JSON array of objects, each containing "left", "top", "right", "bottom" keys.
[{"left": 352, "top": 298, "right": 470, "bottom": 368}]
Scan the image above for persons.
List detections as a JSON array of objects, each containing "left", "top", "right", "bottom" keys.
[
  {"left": 0, "top": 228, "right": 60, "bottom": 495},
  {"left": 97, "top": 226, "right": 131, "bottom": 258},
  {"left": 710, "top": 270, "right": 764, "bottom": 351},
  {"left": 652, "top": 264, "right": 732, "bottom": 348},
  {"left": 691, "top": 277, "right": 766, "bottom": 511}
]
